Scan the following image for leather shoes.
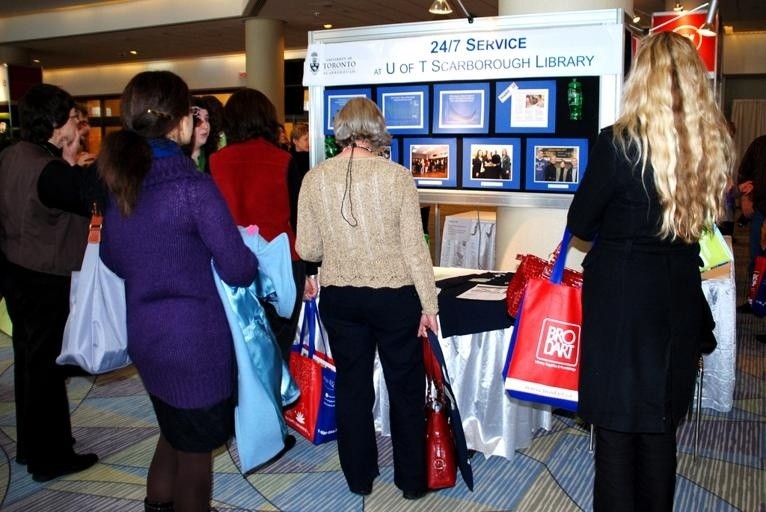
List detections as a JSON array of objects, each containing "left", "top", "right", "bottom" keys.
[{"left": 33, "top": 453, "right": 99, "bottom": 483}]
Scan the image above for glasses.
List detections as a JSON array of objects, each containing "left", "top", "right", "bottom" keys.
[{"left": 189, "top": 105, "right": 199, "bottom": 115}]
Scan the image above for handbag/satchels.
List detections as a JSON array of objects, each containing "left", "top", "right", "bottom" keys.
[
  {"left": 422, "top": 335, "right": 454, "bottom": 489},
  {"left": 503, "top": 222, "right": 584, "bottom": 413},
  {"left": 281, "top": 296, "right": 336, "bottom": 446},
  {"left": 746, "top": 254, "right": 766, "bottom": 316},
  {"left": 506, "top": 235, "right": 584, "bottom": 318},
  {"left": 56, "top": 201, "right": 130, "bottom": 375},
  {"left": 425, "top": 328, "right": 475, "bottom": 492}
]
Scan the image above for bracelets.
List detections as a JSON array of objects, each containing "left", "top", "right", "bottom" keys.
[{"left": 305, "top": 274, "right": 317, "bottom": 279}]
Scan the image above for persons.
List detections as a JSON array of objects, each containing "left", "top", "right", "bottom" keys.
[
  {"left": 718, "top": 120, "right": 753, "bottom": 313},
  {"left": 761, "top": 221, "right": 766, "bottom": 250},
  {"left": 190, "top": 96, "right": 224, "bottom": 173},
  {"left": 412, "top": 155, "right": 448, "bottom": 175},
  {"left": 535, "top": 150, "right": 577, "bottom": 182},
  {"left": 102, "top": 70, "right": 259, "bottom": 512},
  {"left": 473, "top": 148, "right": 510, "bottom": 179},
  {"left": 294, "top": 99, "right": 438, "bottom": 500},
  {"left": 737, "top": 135, "right": 766, "bottom": 279},
  {"left": 568, "top": 32, "right": 739, "bottom": 511},
  {"left": 0, "top": 84, "right": 100, "bottom": 483},
  {"left": 275, "top": 124, "right": 291, "bottom": 149},
  {"left": 210, "top": 87, "right": 318, "bottom": 466},
  {"left": 74, "top": 104, "right": 94, "bottom": 166},
  {"left": 287, "top": 122, "right": 308, "bottom": 236}
]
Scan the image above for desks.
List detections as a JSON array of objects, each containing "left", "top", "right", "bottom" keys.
[{"left": 290, "top": 268, "right": 530, "bottom": 455}]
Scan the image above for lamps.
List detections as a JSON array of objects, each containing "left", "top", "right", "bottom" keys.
[{"left": 428, "top": 4, "right": 473, "bottom": 24}]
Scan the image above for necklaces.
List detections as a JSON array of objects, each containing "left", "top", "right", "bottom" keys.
[{"left": 342, "top": 143, "right": 374, "bottom": 153}]
